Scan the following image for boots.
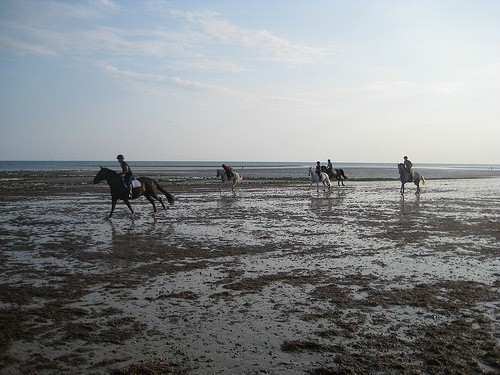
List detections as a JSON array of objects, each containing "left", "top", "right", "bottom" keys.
[{"left": 127, "top": 185, "right": 132, "bottom": 194}]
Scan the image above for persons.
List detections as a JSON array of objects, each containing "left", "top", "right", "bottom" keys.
[
  {"left": 116, "top": 155, "right": 133, "bottom": 198},
  {"left": 222, "top": 164, "right": 234, "bottom": 180},
  {"left": 326, "top": 159, "right": 334, "bottom": 175},
  {"left": 316, "top": 161, "right": 322, "bottom": 180},
  {"left": 404, "top": 156, "right": 412, "bottom": 177}
]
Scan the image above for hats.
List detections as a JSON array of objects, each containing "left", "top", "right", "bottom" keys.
[{"left": 117, "top": 155, "right": 124, "bottom": 159}]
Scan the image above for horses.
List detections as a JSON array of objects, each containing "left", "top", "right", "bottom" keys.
[
  {"left": 93, "top": 166, "right": 176, "bottom": 220},
  {"left": 397, "top": 163, "right": 426, "bottom": 196},
  {"left": 321, "top": 165, "right": 347, "bottom": 187},
  {"left": 308, "top": 167, "right": 332, "bottom": 192},
  {"left": 216, "top": 169, "right": 243, "bottom": 192}
]
